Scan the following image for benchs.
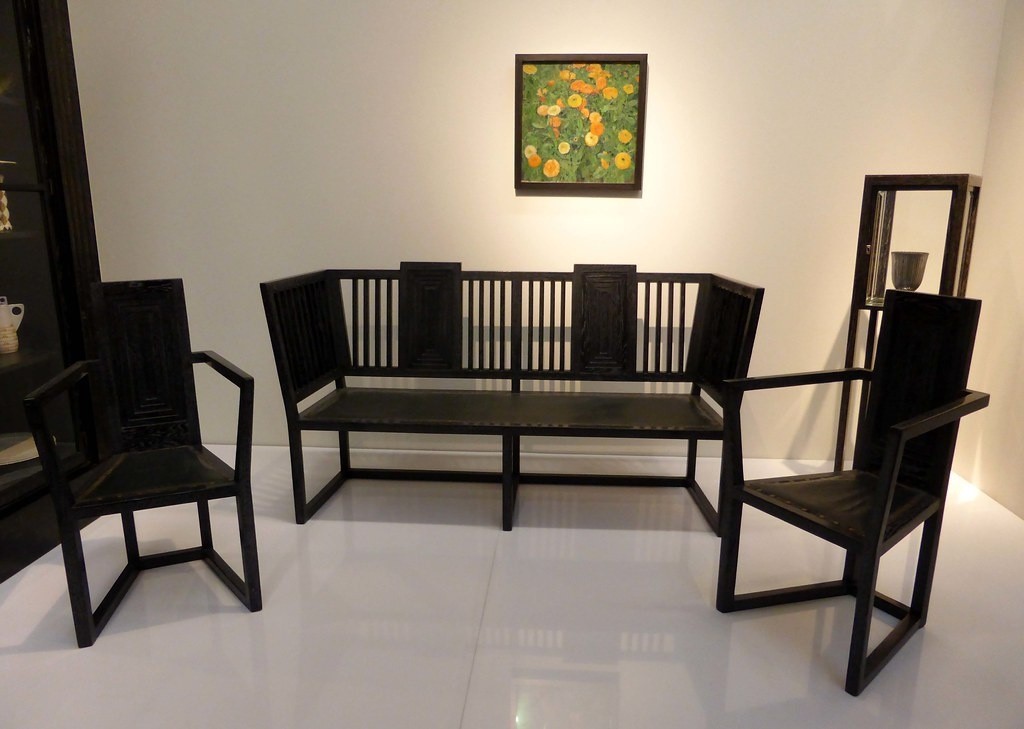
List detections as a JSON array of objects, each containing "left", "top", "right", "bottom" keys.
[{"left": 259, "top": 259, "right": 767, "bottom": 541}]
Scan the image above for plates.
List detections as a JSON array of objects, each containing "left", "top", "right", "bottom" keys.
[{"left": 0, "top": 431, "right": 39, "bottom": 466}]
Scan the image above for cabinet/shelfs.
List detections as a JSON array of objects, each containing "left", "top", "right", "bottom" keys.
[
  {"left": 0, "top": 1, "right": 101, "bottom": 581},
  {"left": 835, "top": 170, "right": 982, "bottom": 471}
]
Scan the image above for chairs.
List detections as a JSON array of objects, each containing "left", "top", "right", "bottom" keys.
[
  {"left": 718, "top": 287, "right": 992, "bottom": 695},
  {"left": 24, "top": 277, "right": 264, "bottom": 650}
]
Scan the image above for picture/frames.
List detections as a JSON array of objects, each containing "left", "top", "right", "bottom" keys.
[{"left": 515, "top": 53, "right": 647, "bottom": 191}]
